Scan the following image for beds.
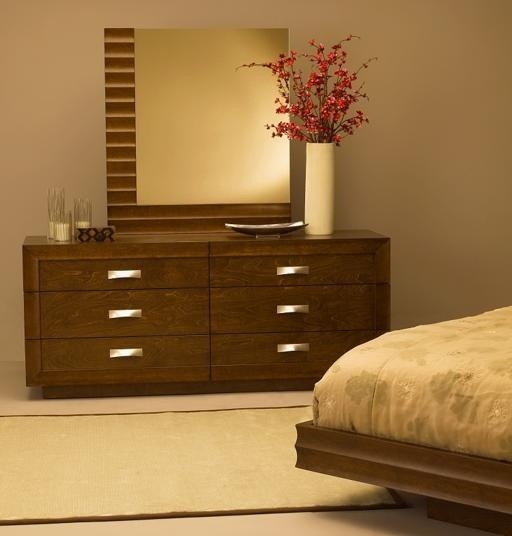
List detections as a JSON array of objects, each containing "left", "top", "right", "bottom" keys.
[{"left": 295, "top": 306, "right": 512, "bottom": 535}]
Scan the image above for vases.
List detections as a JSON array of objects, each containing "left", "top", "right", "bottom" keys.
[{"left": 305, "top": 143, "right": 336, "bottom": 235}]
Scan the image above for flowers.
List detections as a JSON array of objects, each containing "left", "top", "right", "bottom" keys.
[{"left": 235, "top": 35, "right": 379, "bottom": 146}]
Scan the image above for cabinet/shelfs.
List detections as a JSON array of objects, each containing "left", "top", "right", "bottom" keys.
[
  {"left": 210, "top": 239, "right": 390, "bottom": 381},
  {"left": 22, "top": 241, "right": 210, "bottom": 387}
]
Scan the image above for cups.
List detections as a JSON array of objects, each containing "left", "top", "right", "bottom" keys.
[
  {"left": 75, "top": 198, "right": 91, "bottom": 237},
  {"left": 48, "top": 185, "right": 65, "bottom": 239},
  {"left": 55, "top": 209, "right": 72, "bottom": 241}
]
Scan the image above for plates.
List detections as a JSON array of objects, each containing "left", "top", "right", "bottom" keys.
[{"left": 225, "top": 220, "right": 309, "bottom": 238}]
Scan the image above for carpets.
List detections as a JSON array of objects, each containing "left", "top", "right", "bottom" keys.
[{"left": 0, "top": 405, "right": 406, "bottom": 526}]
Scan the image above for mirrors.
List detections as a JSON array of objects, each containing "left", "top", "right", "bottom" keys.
[{"left": 104, "top": 27, "right": 290, "bottom": 231}]
